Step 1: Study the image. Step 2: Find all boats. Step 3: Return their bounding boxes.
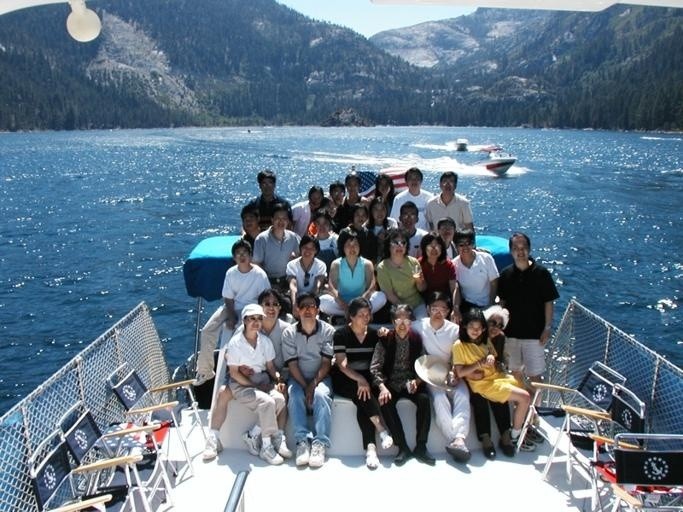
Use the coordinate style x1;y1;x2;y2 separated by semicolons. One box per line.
1;234;683;512
486;152;517;175
457;138;469;150
481;146;503;152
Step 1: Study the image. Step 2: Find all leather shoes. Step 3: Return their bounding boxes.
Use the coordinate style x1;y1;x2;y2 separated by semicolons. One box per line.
395;448;412;463
483;437;496;459
413;445;436;463
498;438;514;457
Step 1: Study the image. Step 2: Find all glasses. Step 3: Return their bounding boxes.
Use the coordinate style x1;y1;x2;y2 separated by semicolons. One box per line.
265;302;279;307
236;250;247;257
301;304;317;310
443;182;454;187
468;326;482;330
335;192;343;196
390;240;408;248
396;319;411;326
427;245;441;249
489;320;504;329
403;214;415;219
247;316;262;320
430;307;448;315
459;241;472;247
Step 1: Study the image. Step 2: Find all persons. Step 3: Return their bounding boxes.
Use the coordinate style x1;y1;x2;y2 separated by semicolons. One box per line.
376;228;428;321
417;231;463;323
337;202;378;265
398;201;429;260
390;167;435;231
318;226;388;325
368;174;396;216
425;171;474;232
192;239;272;387
313;209;341;274
202;288;293;461
330;296;394;469
451;307;545;453
362;196;399;262
369;303;437;464
376;291;472;462
341;174;371;224
247;169;294;232
290;185;324;239
285;234;327;319
306;197;338;235
328;182;350;235
252;203;302;293
464;304;515;458
280;290;336;468
240;204;263;252
450;226;500;316
225;302;285;466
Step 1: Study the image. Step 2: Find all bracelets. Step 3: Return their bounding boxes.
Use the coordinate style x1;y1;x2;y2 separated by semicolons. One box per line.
476;360;482;369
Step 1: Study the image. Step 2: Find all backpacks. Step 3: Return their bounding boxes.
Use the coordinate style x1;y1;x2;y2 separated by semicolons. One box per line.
494;232;561;406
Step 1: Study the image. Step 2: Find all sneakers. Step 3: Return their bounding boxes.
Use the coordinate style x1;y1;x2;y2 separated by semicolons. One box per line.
382;435;393;452
510;435;535;452
365;451;377;469
274;430;292;458
192;370;214;386
258;444;282;465
330;313;343;323
295;442;309;465
242;430;259;455
529;427;544;443
308;440;325;467
204;435;223;460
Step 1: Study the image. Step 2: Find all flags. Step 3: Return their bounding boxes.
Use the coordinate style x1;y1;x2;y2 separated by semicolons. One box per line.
356;170;409;197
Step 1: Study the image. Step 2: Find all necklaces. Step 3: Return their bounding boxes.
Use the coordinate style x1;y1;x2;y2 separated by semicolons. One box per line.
390;258;404;268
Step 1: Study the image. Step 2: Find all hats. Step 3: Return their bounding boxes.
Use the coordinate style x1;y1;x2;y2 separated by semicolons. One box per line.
241;303;267;318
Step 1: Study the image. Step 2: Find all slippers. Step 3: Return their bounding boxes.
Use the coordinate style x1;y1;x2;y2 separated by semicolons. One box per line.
445;440;472;463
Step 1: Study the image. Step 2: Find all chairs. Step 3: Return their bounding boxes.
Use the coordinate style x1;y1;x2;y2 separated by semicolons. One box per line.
563;383;646;512
105;361;208;468
610;433;683;512
28;428;143;512
56;401;177;512
514;361;628;476
